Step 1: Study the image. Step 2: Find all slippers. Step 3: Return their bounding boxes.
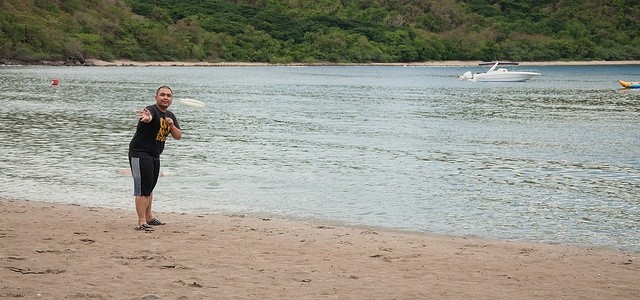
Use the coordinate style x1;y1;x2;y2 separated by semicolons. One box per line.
138;223;154;231
147;217;166;225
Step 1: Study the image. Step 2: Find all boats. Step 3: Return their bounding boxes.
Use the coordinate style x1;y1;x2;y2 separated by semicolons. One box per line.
618;80;640;88
458;62;542;82
178;95;208;110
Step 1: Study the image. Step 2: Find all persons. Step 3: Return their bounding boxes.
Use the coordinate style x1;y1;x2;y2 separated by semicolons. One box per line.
129;86;181;232
